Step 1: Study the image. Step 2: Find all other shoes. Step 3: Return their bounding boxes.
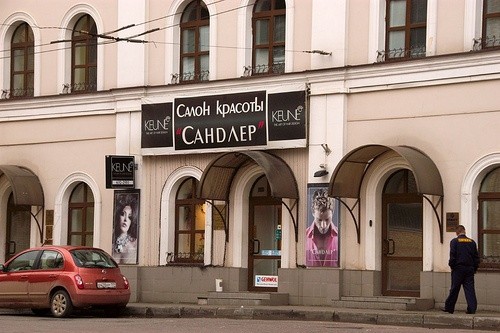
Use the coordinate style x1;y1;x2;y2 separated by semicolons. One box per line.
441;306;453;314
466;311;476;314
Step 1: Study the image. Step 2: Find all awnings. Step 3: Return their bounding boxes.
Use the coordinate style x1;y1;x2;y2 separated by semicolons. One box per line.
196;150;298;242
328;144;443;243
0;165;44;243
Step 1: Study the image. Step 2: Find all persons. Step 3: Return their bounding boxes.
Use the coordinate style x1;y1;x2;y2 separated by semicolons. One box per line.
440;225;478;314
112;202;138;254
305;190;338;266
46;255;55;266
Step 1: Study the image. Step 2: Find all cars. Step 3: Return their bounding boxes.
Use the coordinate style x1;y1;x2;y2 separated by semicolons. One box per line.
0;246;131;318
